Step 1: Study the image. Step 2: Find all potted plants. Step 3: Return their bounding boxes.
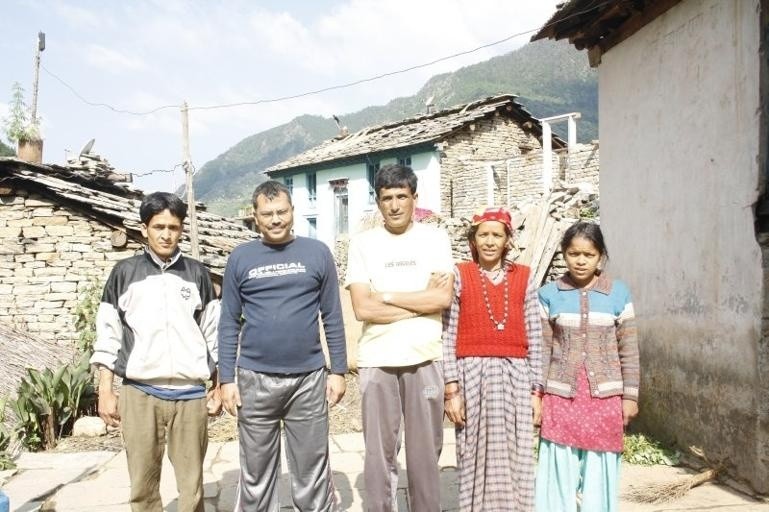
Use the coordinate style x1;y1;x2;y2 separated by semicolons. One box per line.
0;79;43;163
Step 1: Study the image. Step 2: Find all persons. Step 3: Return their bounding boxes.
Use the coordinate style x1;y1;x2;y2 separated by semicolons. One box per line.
219;181;347;512
345;163;454;512
90;190;224;511
442;202;543;511
534;223;641;511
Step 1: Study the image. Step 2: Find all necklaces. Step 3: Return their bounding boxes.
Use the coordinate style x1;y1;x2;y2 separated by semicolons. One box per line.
478;260;512;330
